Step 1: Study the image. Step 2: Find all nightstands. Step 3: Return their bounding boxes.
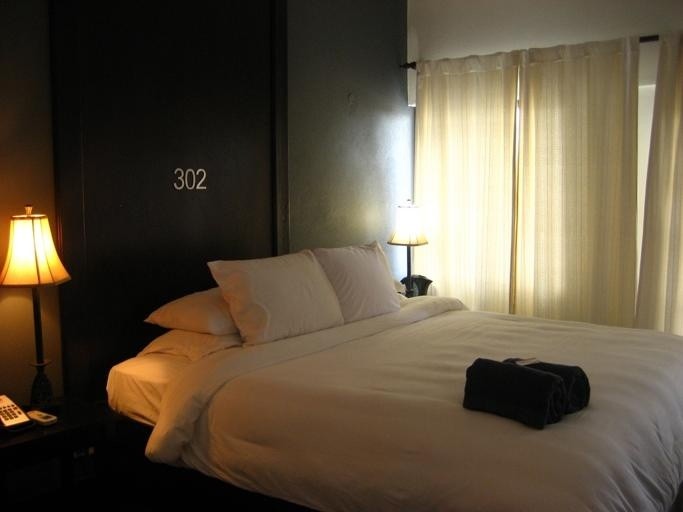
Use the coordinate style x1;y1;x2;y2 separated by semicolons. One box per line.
0;398;129;511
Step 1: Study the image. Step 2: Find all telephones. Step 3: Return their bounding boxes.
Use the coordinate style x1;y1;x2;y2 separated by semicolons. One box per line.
0;394;30;427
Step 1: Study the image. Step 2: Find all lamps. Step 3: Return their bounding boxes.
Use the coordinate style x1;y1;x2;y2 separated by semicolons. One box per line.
386;197;430;297
0;204;72;407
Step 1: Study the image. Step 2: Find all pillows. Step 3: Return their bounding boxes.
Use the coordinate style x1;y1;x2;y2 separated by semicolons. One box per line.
207;249;345;348
136;329;242;364
312;239;401;324
143;285;237;336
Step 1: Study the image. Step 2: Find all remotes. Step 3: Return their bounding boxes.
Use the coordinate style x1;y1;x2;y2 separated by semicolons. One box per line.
26;410;58;426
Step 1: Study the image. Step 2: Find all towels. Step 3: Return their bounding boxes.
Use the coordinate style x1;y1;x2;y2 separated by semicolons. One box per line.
463;358;590;429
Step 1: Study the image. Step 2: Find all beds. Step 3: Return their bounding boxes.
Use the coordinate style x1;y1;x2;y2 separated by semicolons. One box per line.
107;295;683;512
